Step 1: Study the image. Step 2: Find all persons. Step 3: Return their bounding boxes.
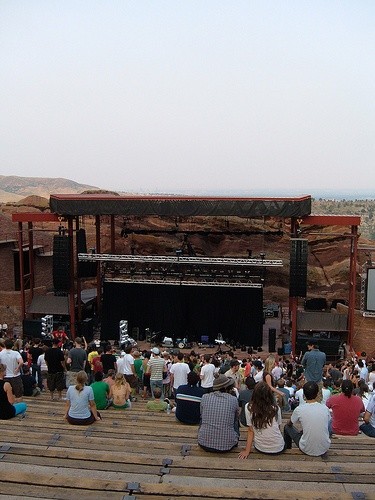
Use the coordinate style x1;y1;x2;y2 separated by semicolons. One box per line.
262;354;283;435
360;381;375;438
63;370;101;425
301;338;326;402
145;347;167;398
147;388;168;411
108;373;132;409
169;352;191;391
283;381;333;457
199;353;215;394
326;380;365;436
102;368;116;387
224;359;241;392
237;382;285;459
90;370;112;410
196;374;241;453
175;371;205;426
120;346;138;388
0;327;375;459
65;336;87;372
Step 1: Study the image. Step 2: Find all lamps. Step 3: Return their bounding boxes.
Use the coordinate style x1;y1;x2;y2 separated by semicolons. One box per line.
41;317;48;336
120;320;129;341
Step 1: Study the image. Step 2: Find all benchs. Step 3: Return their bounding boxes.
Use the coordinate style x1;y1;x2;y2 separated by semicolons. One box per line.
0;387;375;500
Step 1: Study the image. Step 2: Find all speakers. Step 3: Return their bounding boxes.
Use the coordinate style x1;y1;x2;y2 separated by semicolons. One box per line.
52;235;73;291
288;238;308;297
269;328;276;353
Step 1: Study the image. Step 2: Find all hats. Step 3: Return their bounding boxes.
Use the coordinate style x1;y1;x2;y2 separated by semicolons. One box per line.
230;361;240;366
151;347;160;354
212;374;235;390
252;360;263;368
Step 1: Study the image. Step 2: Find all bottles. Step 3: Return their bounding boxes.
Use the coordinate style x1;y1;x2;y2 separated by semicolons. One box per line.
167;404;170;415
127;399;131;408
